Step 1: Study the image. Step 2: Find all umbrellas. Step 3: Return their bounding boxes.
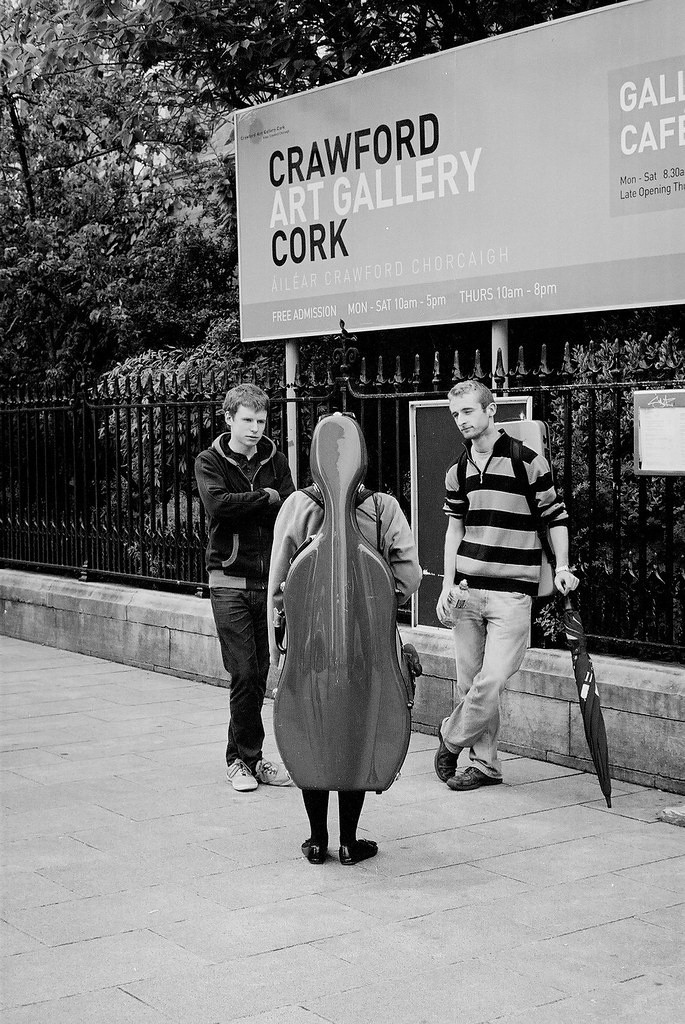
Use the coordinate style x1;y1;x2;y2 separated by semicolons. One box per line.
561;584;612;809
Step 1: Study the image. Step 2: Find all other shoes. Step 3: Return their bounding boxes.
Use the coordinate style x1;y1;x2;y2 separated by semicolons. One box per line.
339;839;379;866
301;838;328;863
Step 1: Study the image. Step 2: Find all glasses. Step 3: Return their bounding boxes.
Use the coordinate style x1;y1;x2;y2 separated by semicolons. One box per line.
318;412;356;421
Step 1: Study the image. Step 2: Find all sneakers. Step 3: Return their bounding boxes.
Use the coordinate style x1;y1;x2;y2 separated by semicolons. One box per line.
447;763;503;791
254;756;293;787
226;758;258;791
434;724;459;782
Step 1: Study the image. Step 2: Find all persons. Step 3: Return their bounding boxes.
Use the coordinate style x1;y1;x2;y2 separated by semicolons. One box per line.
266;412;421;864
194;384;297;791
435;380;579;791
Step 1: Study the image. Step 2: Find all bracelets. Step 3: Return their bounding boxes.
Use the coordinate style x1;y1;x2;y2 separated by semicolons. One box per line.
555;565;570;573
